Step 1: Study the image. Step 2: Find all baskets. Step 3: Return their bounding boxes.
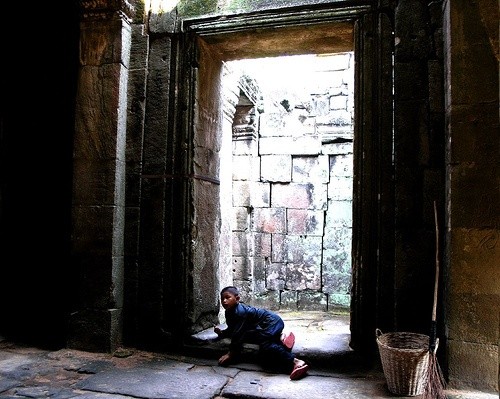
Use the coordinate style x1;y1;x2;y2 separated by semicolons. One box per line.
376;327;439;397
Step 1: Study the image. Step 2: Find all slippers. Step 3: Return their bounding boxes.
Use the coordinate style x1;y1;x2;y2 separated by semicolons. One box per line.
283;331;295;349
290;363;308;379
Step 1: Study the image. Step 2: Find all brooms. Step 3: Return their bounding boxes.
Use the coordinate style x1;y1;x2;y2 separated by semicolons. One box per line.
408;200;454;399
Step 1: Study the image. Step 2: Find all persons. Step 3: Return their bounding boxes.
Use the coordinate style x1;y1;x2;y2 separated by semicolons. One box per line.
213;286;308;380
